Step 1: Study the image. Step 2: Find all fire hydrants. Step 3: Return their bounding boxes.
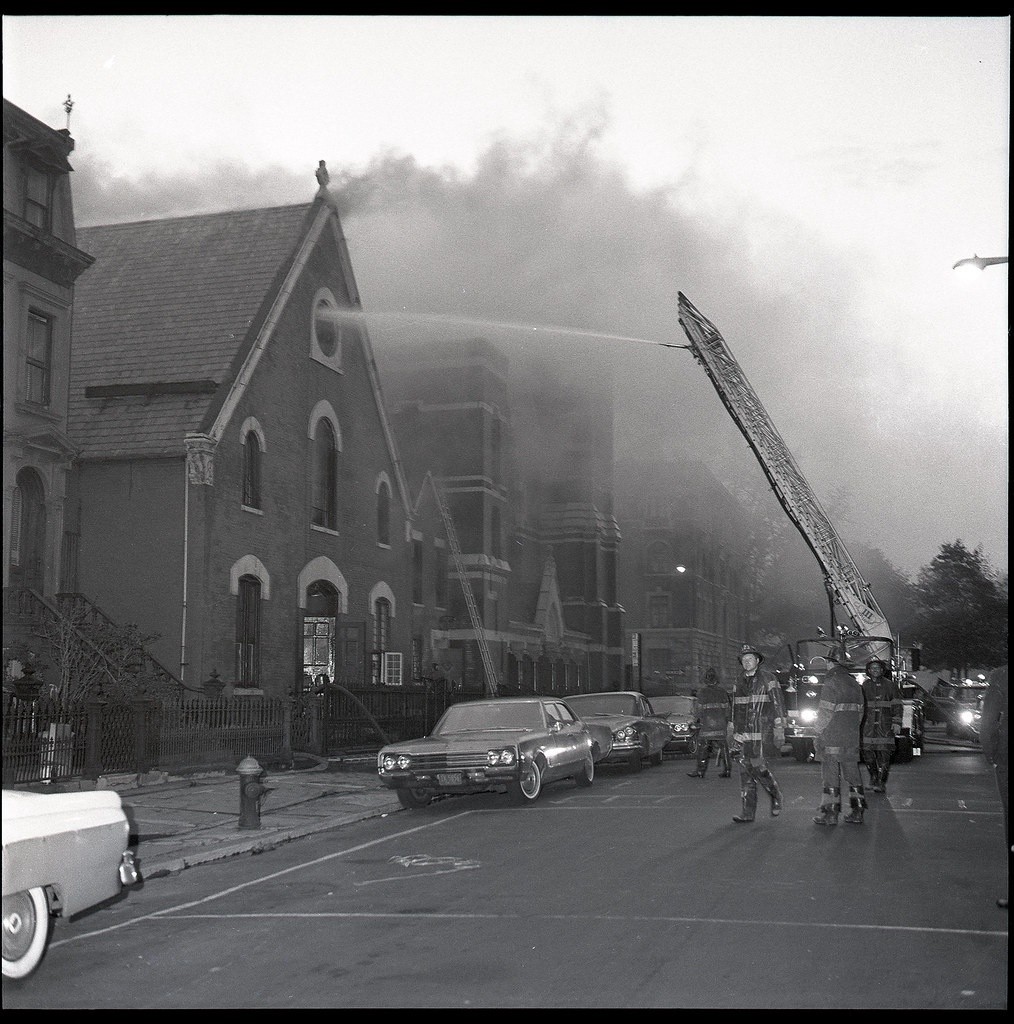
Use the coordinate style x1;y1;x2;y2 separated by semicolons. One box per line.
237;754;269;831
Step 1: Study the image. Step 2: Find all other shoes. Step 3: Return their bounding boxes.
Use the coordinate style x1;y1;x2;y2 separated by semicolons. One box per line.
996;899;1008;907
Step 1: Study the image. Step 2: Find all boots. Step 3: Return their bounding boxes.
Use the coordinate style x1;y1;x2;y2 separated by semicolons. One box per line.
865;760;878;790
873;759;891;793
686;758;708;778
732;782;758;822
843;786;868;823
813;787;841;825
719;755;732;777
763;780;784;816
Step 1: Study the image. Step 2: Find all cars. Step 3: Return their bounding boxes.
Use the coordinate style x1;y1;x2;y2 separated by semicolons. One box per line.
561;692;670;773
376;697;613;815
0;785;139;985
944;680;989;738
645;696;703;756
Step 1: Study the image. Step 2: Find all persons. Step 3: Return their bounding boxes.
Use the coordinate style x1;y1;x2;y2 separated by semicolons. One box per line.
813;646;866;825
687;664;733;778
860;657;904;794
728;642;782;822
980;664;1008;910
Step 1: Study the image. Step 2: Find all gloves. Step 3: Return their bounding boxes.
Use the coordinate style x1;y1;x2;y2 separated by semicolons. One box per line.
891;723;901;734
773;733;785;748
813;735;825;751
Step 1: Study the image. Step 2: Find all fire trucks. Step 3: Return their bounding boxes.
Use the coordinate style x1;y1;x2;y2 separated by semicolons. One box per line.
657;292;927;766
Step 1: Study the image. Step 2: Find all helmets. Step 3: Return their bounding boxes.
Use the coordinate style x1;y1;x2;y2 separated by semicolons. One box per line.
821;646;854;667
738;643;764;666
866;657;887;676
